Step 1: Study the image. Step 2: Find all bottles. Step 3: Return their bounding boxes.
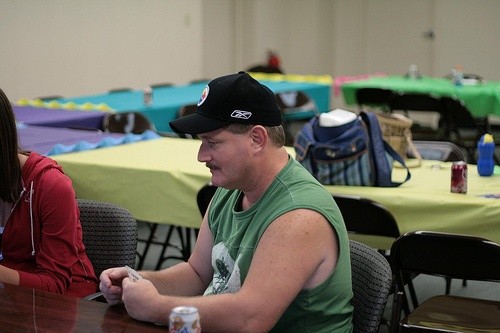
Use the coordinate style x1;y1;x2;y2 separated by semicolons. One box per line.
477;134;495;176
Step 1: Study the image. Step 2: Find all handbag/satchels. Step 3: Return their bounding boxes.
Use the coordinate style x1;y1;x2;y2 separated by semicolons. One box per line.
365;110;423;168
294;111;411;188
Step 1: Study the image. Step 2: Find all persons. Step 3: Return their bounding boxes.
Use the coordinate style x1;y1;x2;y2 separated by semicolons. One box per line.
99;71;354;333
0;89;97;300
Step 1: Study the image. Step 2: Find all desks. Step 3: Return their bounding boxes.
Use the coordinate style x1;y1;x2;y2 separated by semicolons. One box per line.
17;73;332;136
12;106;160;157
341;75;500;117
0;280;169;333
48;137;500;262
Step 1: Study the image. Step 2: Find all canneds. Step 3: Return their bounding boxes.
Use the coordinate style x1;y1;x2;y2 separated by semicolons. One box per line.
407;64;418;80
168;306;201;333
450;161;468;194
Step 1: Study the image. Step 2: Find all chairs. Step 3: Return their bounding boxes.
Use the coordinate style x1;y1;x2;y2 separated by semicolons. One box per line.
406;141;467;295
354;88;500;166
330;194;420;309
74;198;138;303
391;230;500;333
98;112;184;270
196;182;218;219
348;240;393;333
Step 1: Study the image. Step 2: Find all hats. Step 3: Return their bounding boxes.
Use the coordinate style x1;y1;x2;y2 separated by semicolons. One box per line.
169;71;283;135
